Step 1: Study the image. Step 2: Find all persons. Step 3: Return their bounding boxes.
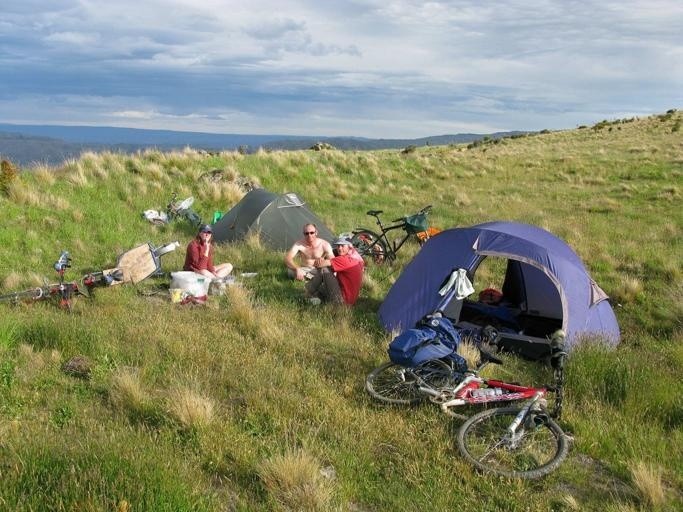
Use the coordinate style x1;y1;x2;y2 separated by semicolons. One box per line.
184;224;234;295
302;238;364;309
284;224;335;282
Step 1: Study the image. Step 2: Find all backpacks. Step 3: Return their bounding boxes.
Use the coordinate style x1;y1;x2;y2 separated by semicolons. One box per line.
386;315;457;366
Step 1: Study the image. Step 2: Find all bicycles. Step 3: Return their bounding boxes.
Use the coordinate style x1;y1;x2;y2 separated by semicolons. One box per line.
165;192;201;237
348;205;432;269
365;328;567;480
0;251;123;314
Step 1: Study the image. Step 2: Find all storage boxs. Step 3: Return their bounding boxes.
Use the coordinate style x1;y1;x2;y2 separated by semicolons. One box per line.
169;269;212;296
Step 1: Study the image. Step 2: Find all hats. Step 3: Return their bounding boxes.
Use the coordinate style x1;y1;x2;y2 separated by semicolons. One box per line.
199;225;212;234
332;234;351;245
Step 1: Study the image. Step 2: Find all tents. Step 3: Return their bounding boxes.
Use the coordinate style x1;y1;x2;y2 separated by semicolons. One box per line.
211;188;339;255
376;220;621;371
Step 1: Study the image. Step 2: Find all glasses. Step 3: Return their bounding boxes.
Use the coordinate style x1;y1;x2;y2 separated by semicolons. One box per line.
305;230;314;237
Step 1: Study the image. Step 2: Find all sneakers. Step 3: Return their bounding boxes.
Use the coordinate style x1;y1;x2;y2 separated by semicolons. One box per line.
301;296;321;305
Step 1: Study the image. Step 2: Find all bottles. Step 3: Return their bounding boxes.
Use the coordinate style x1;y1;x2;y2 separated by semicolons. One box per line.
454;375;482;400
470;386;511;397
